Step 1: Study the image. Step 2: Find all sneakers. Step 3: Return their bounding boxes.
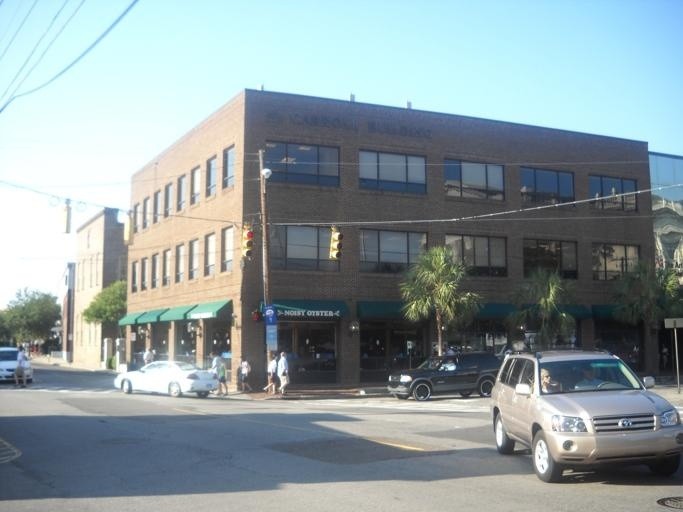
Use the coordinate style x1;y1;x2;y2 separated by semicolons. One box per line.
224;393;229;395
263;388;268;392
15;384;19;386
273;391;277;395
282;392;287;395
21;385;26;388
241;390;246;393
215;392;221;395
249;389;253;391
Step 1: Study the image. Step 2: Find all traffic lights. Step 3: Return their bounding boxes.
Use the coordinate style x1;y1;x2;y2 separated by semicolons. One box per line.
240;225;255;257
327;227;343;261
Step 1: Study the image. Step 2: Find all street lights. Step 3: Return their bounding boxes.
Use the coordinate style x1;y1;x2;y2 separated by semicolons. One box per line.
257;167;277;397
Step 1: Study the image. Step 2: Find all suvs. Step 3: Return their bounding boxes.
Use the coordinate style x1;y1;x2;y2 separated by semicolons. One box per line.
487;345;682;486
384;349;502;402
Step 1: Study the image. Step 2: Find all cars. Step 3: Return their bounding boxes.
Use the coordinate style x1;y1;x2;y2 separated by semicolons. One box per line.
0;345;34;385
113;359;219;400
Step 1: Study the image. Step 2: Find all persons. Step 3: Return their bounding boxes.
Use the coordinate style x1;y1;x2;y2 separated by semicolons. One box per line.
143;348;153;365
239;355;251;393
209;351;222;395
572;366;605;390
277;351;288;394
152;347;159;362
661;343;670;369
261;354;278;394
530;367;560;394
13;346;27;387
216;358;228;396
441;359;456;371
